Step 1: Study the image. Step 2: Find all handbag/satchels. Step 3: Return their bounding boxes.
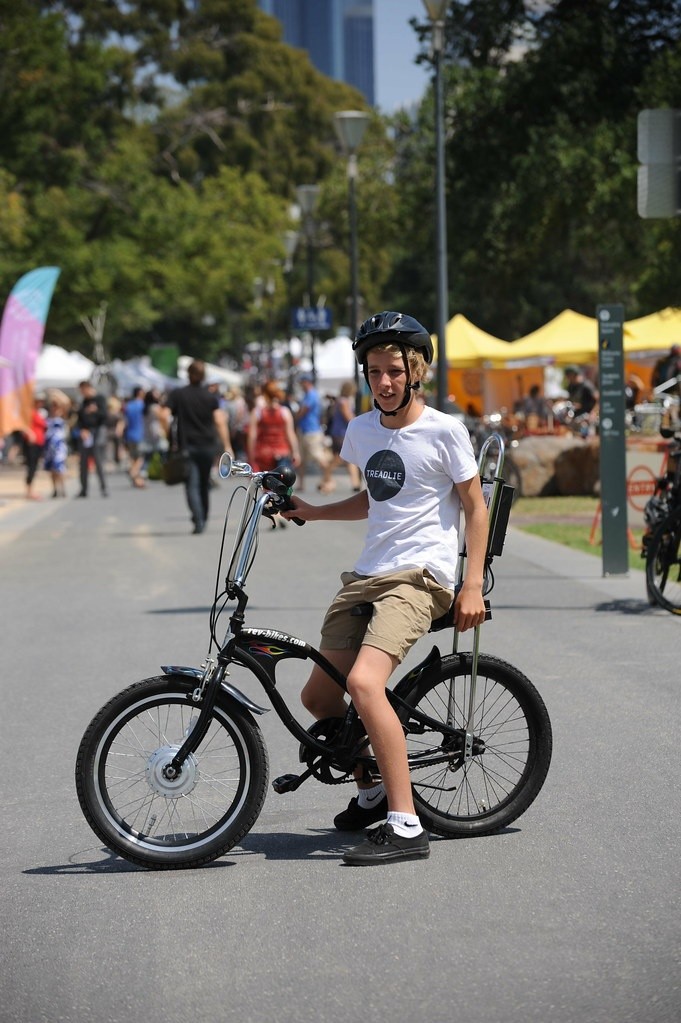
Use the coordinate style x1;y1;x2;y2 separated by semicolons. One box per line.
162;449;191;485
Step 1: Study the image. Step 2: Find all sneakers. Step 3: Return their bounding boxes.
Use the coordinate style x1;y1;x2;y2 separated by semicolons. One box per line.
333;795;385;830
343;823;430;866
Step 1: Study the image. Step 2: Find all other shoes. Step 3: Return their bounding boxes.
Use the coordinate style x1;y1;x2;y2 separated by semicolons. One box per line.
194;520;204;534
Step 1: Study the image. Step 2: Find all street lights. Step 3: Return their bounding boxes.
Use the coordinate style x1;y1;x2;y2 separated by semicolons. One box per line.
331;110;370;415
280;186;324;388
423;1;455;414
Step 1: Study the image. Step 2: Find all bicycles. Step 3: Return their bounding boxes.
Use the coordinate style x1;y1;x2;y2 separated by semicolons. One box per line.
74;433;555;869
639;430;681;615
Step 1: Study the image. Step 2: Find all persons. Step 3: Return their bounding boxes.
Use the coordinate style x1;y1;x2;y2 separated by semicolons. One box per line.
281;312;489;866
650;345;681;408
0;372;363;500
157;361;235;533
514;385;545;414
564;365;597;416
248;381;301;530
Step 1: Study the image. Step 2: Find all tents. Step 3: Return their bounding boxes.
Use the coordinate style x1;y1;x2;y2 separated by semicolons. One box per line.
289;336;366;388
30;344;243;393
423;307;681;417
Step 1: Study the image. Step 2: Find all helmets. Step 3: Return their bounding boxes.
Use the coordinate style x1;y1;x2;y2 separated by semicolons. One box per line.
352;312;433;365
